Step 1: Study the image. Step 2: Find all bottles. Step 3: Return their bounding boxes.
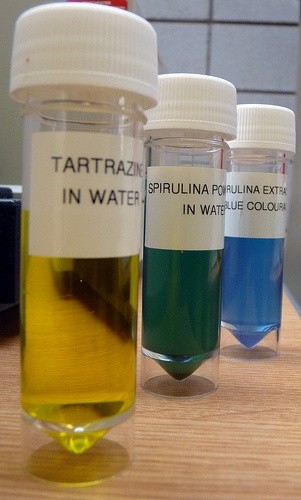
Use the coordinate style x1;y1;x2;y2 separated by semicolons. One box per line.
222;104;296;360
127;66;237;397
9;0;161;487
